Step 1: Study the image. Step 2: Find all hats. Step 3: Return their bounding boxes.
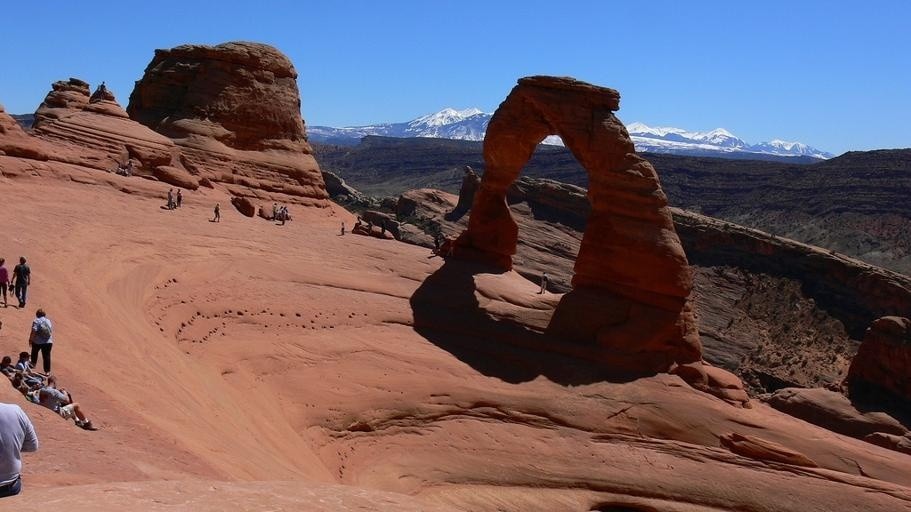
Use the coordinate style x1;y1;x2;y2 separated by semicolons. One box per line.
19;352;31;357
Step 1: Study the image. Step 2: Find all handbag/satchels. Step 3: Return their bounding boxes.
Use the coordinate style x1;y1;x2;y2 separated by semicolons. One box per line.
8;283;15;293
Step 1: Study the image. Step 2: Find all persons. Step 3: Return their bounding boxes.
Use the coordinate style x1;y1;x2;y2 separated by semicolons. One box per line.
168;188;182;209
0;308;97;431
357;216;386;236
434;238;440;256
0;401;38;497
341;223;344;235
541;273;547;294
0;257;31;307
273;203;288;225
214;203;220;222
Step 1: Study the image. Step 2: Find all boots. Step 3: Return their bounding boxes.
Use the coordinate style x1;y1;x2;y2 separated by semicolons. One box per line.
75;421;98;431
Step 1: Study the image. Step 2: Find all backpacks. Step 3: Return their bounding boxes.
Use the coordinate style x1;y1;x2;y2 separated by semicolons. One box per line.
544;277;547;281
38;320;50;340
